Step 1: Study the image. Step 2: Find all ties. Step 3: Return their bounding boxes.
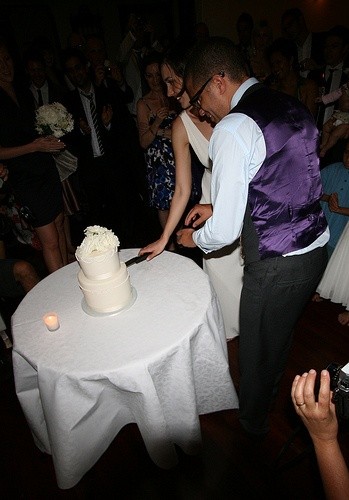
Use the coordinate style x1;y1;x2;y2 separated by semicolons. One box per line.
80;91;104;155
36;89;44;107
317;68;339;131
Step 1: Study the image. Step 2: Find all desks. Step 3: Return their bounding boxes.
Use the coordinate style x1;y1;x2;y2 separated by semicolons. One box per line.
10;249;240;490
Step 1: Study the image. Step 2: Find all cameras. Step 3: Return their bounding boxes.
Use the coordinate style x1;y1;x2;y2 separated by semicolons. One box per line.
325;362;349;416
103;64;111;75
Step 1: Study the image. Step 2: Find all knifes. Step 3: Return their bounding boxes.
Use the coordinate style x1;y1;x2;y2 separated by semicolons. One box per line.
125;252;153;267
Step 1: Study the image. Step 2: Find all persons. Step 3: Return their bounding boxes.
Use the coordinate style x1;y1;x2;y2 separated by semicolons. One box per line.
0;22;349;369
290;367;349;500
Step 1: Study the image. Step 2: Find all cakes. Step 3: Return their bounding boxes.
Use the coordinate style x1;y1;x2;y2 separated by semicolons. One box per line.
75;225;133;313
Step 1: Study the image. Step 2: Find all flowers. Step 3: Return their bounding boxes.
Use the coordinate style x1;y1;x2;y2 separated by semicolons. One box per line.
36;103;73;141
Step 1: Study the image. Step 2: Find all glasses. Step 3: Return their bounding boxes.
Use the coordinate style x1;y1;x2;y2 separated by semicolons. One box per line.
189;72;225;110
